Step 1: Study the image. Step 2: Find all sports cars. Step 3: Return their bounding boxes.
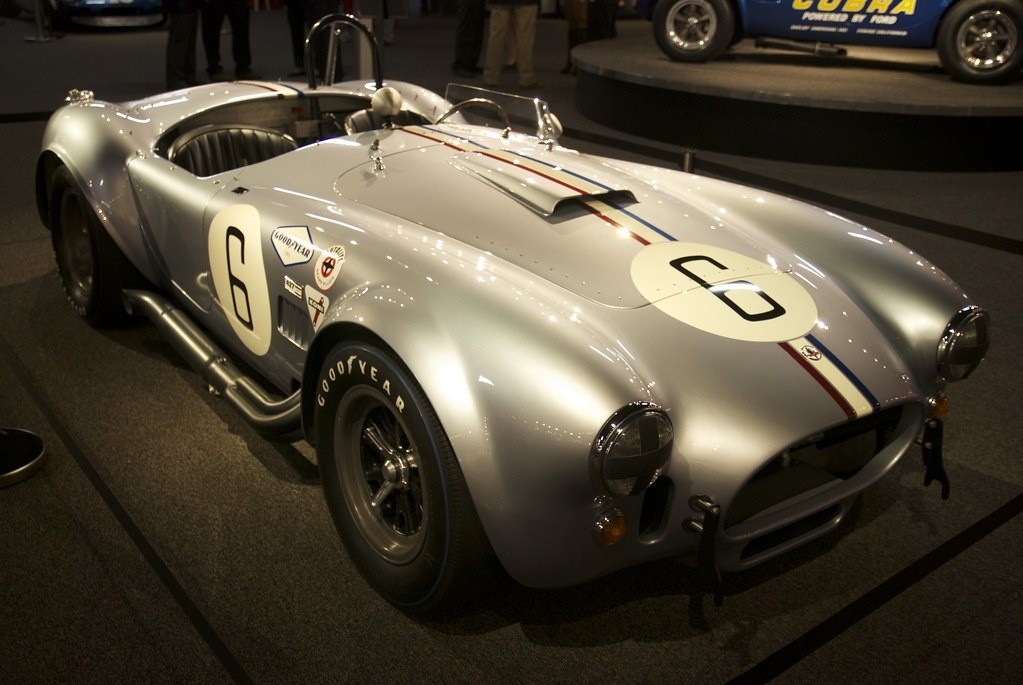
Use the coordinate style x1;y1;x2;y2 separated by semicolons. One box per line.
33;9;989;624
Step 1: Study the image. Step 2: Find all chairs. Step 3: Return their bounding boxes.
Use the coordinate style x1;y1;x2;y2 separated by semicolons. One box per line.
344;106;434;135
165;122;299;178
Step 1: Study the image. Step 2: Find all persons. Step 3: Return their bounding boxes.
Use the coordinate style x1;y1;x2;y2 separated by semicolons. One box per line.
159;0;257;96
452;0;618;96
284;1;329;78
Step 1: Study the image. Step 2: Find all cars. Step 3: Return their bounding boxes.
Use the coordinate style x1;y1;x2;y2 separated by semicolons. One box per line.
599;0;1023;87
